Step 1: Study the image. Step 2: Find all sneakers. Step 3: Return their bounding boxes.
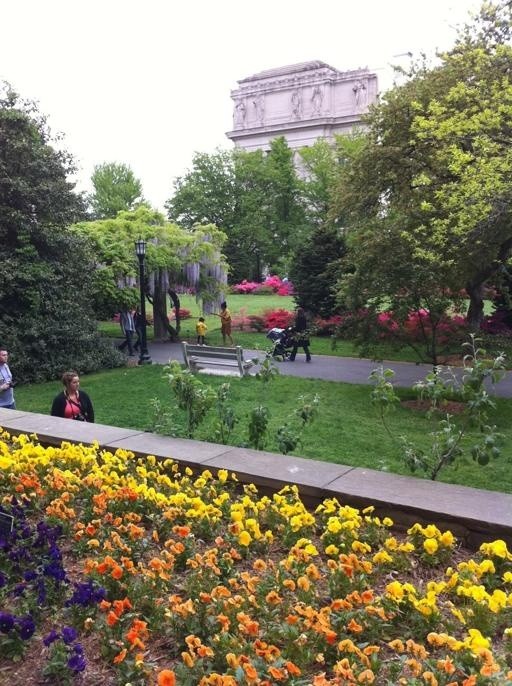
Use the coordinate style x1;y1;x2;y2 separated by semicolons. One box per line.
118;344;138;355
287;355;294;360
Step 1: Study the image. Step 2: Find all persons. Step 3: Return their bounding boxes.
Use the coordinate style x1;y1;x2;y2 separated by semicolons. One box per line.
233;79;369;129
0;347;18;410
131;304;152;353
117;308;137;356
210;301;234;348
284;307;312;362
51;370;95;425
195;317;209;347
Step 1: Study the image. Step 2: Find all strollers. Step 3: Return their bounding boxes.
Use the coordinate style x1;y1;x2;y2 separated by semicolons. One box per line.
266;327;294;362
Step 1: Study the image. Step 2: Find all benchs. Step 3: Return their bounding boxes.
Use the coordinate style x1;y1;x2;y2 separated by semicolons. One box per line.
181;341;253;378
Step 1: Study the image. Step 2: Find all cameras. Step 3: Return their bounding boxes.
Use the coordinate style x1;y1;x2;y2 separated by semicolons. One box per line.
8;379;19;389
74;412;85;421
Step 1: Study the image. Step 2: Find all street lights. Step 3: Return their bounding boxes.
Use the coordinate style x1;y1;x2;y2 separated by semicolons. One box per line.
134;235;152;365
